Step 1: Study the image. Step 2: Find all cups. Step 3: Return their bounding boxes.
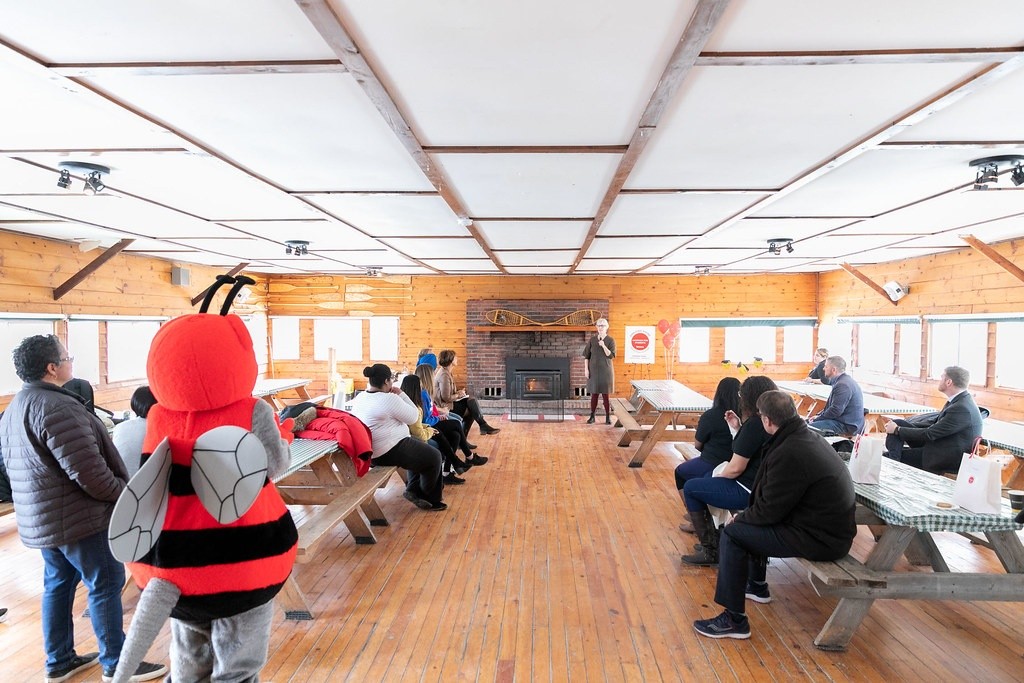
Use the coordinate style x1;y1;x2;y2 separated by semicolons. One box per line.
1008;489;1024;512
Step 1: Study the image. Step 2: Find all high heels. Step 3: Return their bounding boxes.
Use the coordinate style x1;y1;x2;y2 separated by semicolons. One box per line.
458;441;477;450
480;423;500;435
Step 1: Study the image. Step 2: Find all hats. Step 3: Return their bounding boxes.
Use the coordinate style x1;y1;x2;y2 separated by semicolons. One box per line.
596;318;608;326
416;354;437;371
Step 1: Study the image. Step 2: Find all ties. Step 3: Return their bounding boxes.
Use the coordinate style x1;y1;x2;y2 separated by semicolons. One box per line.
940;401;949;413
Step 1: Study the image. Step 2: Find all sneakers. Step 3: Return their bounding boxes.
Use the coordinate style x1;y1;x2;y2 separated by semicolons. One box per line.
102;657;167;683
428;500;447;511
742;579;772;604
48;652;101;683
443;473;466;484
465;453;488;466
403;485;432;509
694;609;751;640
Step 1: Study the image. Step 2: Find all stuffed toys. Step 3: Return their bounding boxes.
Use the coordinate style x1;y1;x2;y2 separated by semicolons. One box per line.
107;314;298;683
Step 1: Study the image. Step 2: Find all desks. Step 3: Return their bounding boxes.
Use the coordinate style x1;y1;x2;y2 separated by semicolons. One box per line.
616;377;714;467
79;437;389;620
251;378;313;410
769;380;1024;650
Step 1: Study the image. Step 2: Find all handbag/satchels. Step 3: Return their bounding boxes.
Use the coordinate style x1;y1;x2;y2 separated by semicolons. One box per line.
952;437;1001;515
822;435;854;461
848;420;883;485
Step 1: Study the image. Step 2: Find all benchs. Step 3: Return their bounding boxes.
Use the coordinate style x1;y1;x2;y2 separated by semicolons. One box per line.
278;466;400;563
674;441;888;598
274;394;332;419
610;397;642;442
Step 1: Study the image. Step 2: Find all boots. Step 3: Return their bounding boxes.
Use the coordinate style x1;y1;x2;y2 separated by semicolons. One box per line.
587;413;596;423
606;414;611;424
680;507;720;569
447;448;472;475
677;489;699;537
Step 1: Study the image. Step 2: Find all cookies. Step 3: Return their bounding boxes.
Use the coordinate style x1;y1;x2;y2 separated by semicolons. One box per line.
936;502;951;508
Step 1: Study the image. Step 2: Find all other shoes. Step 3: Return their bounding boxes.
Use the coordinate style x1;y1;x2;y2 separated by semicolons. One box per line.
0;608;9;623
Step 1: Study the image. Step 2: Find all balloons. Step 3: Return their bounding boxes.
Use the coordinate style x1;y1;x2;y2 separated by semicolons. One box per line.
662;333;674;350
658;319;669;334
669;321;680;337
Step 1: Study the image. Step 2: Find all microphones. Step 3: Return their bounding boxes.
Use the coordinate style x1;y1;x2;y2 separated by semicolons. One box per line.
599;334;602;340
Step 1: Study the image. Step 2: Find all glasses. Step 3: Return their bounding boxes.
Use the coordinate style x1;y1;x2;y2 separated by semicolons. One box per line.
757;412;763;418
738;390;742;398
388;374;395;380
57;352;74;363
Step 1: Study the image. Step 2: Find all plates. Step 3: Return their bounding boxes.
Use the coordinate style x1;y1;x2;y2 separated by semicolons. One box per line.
930;502;960;510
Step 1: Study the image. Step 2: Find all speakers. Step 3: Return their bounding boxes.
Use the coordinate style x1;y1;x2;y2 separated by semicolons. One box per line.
171;267;191;286
233;288;252;304
883;281;904;302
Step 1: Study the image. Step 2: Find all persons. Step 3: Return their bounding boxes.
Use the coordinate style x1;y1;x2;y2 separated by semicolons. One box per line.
692;391;856;639
401;347;499;486
0;334;168;683
680;376;779;567
884;366;982;474
806;356;864;436
803;347;831;384
674;377;743;533
111;386;158;479
350;364;447;511
582;318;616;425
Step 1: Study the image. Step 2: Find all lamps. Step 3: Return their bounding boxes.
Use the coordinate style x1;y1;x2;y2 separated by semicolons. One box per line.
284;240;309;256
969;155;1024;190
695;266;712;275
56;159;111;195
365;267;382;277
768;238;794;255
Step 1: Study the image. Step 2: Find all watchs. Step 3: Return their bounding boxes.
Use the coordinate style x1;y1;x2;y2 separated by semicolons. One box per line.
894;427;899;436
